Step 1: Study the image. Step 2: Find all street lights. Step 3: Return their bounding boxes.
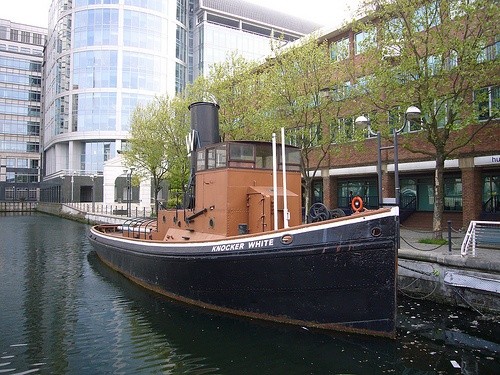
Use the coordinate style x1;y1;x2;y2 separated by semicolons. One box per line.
90;174;98;212
353;106;421;248
122;166;136;218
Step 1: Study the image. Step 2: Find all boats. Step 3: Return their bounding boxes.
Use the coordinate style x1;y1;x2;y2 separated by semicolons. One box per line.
88;99;402;341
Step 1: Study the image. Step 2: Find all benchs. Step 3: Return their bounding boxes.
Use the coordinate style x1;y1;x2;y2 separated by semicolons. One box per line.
101;226;118;233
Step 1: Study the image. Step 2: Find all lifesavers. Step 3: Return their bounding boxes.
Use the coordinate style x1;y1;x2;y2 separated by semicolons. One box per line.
352;196;363;211
